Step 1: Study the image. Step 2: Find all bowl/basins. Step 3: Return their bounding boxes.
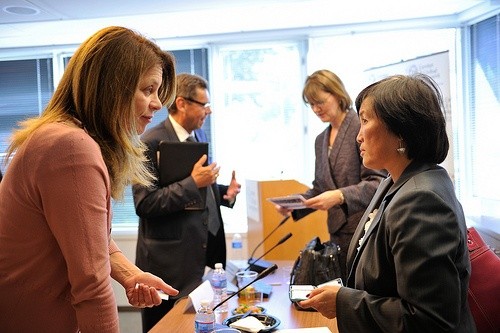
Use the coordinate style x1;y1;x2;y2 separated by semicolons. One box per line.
211;328;241;333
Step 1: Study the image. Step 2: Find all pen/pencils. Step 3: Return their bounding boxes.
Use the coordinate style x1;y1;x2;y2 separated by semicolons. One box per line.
270;283;281;286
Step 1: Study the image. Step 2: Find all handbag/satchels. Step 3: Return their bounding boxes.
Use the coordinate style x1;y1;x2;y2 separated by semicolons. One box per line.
289;236;343;312
467;226;500;333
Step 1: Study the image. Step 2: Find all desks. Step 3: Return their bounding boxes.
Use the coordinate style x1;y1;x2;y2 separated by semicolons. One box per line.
145;258;339;333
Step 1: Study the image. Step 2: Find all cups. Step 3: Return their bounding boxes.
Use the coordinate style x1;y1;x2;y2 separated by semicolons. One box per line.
245;283;263;307
236;271;258;307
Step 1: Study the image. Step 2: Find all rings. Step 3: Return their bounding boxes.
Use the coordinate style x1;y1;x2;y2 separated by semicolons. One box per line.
215;174;218;178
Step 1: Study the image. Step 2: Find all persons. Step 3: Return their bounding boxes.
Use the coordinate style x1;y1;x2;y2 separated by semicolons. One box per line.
296;76;473;333
132;72;240;333
275;70;389;278
0;26;179;333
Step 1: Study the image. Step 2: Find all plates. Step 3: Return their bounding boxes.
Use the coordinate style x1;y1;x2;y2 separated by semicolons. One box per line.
222;313;281;333
231;307;266;315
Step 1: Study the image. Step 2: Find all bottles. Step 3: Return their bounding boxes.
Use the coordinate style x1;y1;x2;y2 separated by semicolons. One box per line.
231;233;242;259
194;301;217;333
211;263;228;313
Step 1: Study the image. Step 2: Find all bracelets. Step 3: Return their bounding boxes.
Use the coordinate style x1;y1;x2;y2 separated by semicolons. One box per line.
335;189;345;206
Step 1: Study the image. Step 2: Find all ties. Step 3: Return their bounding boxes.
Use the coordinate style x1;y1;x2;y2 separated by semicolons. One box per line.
186;136;220;236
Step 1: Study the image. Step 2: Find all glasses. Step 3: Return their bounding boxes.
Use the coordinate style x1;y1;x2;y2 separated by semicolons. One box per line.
176;96;210;107
308;93;333;108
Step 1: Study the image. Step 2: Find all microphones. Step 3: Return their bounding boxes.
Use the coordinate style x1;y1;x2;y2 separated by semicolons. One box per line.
248;215;290;272
237;233;292;298
212;264;278;311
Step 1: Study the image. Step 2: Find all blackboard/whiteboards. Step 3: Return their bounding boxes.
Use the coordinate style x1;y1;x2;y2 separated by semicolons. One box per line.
364;50;452;191
